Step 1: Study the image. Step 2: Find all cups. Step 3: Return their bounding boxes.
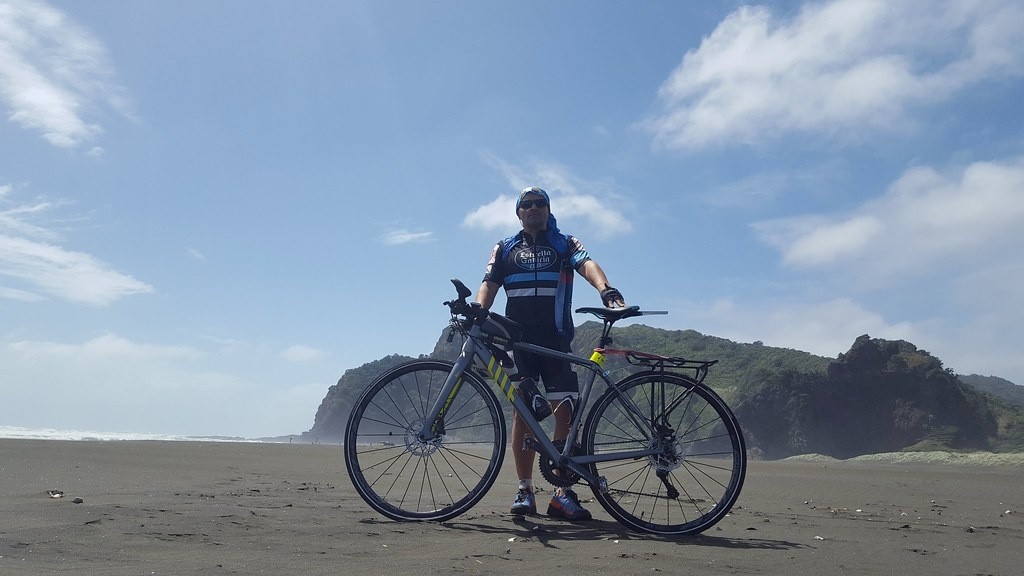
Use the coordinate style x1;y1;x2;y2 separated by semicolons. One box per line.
521;378;553;423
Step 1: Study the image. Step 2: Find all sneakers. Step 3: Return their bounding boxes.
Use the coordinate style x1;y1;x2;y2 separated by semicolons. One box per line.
510;488;537;516
547;491;591;522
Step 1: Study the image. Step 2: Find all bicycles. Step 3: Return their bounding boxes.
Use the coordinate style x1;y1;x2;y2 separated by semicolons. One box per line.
342;279;748;536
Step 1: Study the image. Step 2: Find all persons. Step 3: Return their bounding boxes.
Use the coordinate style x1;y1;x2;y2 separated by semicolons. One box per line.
468;187;625;520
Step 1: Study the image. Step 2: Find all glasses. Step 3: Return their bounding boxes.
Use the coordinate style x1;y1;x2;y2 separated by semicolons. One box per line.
520;199;546;209
